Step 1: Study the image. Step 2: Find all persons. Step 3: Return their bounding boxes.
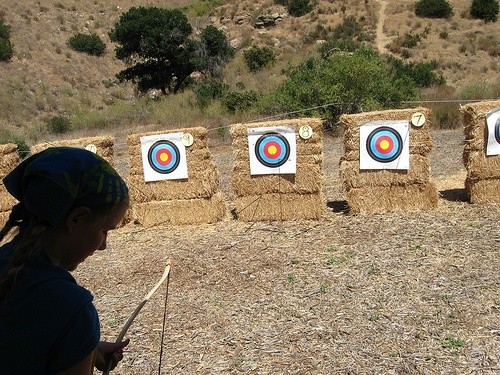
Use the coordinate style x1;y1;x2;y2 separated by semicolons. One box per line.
0;146;131;375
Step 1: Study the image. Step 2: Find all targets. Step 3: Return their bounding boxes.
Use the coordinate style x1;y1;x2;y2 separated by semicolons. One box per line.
140;132;190;184
359;119;410;171
246;126;298;176
485;108;500;157
